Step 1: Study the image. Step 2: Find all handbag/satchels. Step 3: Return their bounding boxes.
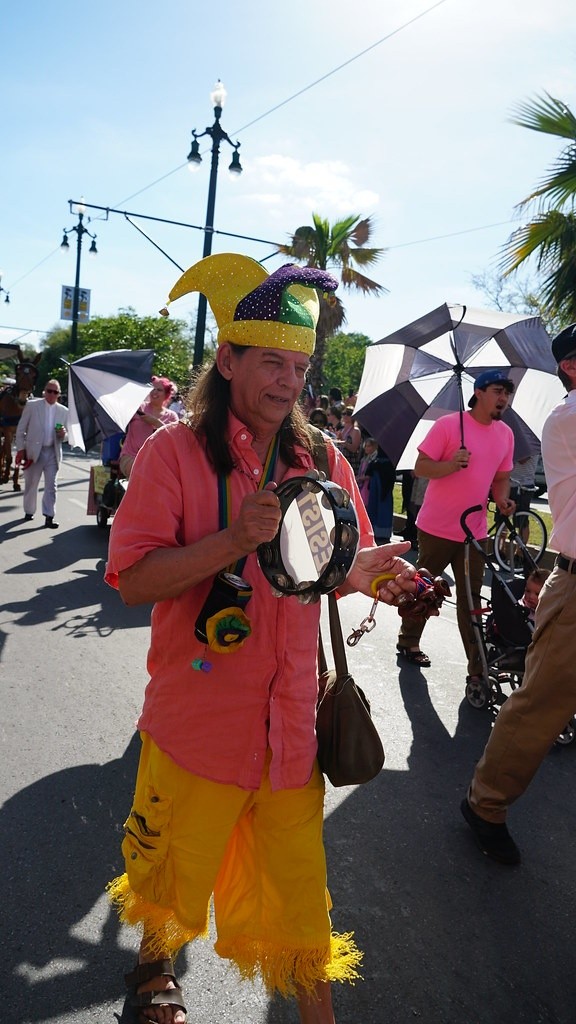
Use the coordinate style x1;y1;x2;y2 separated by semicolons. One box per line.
316;669;384;788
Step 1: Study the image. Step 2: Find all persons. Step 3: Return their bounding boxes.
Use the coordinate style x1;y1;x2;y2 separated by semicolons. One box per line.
103;254;417;1024
304;388;430;550
524;569;552;620
488;453;540;564
166;383;196;418
15;380;69;528
396;370;516;682
460;323;576;864
118;376;179;482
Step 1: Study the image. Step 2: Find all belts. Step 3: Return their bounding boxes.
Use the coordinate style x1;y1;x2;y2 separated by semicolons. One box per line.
558;553;576;574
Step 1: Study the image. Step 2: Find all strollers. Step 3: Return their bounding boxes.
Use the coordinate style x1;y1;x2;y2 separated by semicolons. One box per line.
459;503;576;748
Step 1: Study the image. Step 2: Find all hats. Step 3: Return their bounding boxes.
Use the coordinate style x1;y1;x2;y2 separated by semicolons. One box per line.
467;370;508;409
552;322;576;364
166;253;339;356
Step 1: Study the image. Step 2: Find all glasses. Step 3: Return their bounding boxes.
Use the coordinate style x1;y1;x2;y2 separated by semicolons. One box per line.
314;419;325;424
46;388;60;395
342;412;352;417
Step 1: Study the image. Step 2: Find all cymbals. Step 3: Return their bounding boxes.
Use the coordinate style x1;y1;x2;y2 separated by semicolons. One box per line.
256;469;359;606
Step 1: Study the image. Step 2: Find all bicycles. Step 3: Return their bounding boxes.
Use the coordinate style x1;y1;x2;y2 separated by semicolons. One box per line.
488;470;547;573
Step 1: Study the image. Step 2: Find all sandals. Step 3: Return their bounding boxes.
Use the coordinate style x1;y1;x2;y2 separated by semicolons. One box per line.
123;945;187;1024
398;641;431;665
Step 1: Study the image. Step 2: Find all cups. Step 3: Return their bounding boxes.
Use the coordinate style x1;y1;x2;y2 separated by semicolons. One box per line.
56;424;63;431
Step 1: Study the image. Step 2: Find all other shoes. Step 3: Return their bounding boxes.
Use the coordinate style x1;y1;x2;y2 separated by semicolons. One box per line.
498;557;525;573
45;517;59;528
461;797;521;868
404;539;419;552
486;550;507;564
394;528;408;537
26;513;34;521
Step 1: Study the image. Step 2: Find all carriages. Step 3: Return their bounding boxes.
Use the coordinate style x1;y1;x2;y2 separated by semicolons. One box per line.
0;343;43;492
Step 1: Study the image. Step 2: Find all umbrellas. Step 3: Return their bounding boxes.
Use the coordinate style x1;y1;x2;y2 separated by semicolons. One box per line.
351;302;568;471
60;348;156;457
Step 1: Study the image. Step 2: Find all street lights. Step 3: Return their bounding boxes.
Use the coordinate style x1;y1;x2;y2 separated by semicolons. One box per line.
61;196;99;362
187;77;242;375
